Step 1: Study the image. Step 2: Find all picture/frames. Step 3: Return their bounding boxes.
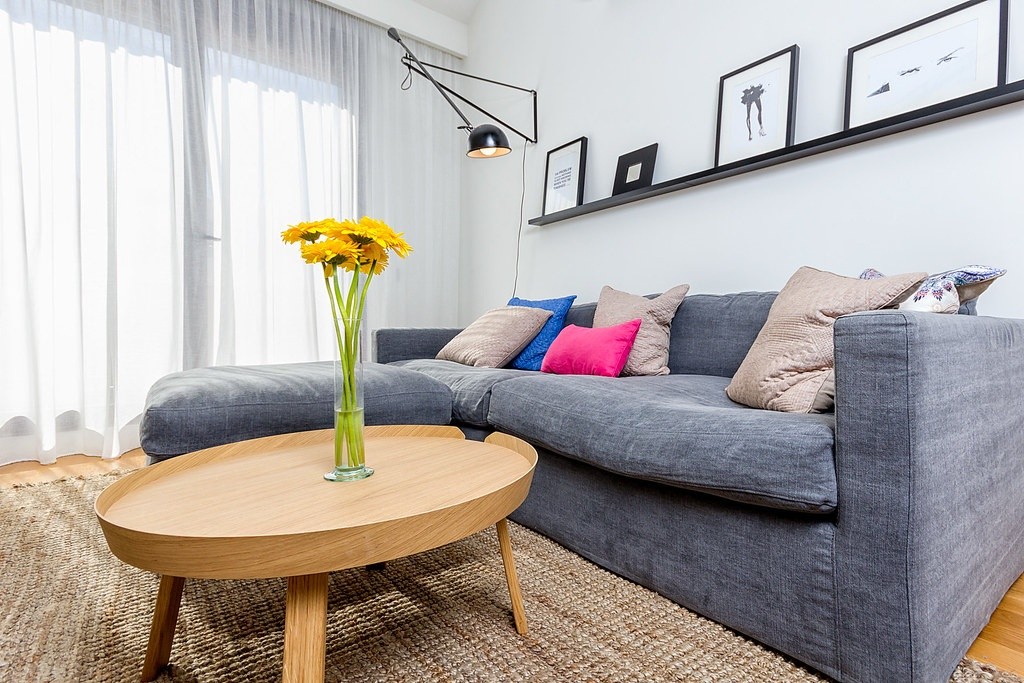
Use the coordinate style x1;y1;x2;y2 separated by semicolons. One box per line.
844;0;1008;131
542;136;588;216
714;44;800;168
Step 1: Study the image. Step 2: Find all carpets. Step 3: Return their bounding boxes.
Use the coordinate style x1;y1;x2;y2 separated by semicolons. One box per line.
0;465;1024;683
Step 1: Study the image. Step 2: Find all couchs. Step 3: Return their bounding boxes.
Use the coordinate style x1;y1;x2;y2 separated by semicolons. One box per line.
140;294;1023;683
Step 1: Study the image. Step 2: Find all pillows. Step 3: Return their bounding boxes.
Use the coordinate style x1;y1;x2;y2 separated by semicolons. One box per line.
592;284;690;376
506;295;578;371
857;264;1007;315
435;305;554;368
540;318;642;378
725;265;929;413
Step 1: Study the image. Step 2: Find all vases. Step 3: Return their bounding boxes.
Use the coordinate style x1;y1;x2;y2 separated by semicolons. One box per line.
323;318;378;483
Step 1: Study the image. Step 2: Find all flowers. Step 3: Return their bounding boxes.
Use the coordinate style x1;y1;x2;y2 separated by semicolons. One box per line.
280;216;413;318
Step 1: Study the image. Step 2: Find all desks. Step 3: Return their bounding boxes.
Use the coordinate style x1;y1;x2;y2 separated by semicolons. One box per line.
93;424;540;683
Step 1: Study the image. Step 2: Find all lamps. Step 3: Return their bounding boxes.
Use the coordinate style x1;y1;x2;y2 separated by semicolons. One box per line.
387;27;537;159
